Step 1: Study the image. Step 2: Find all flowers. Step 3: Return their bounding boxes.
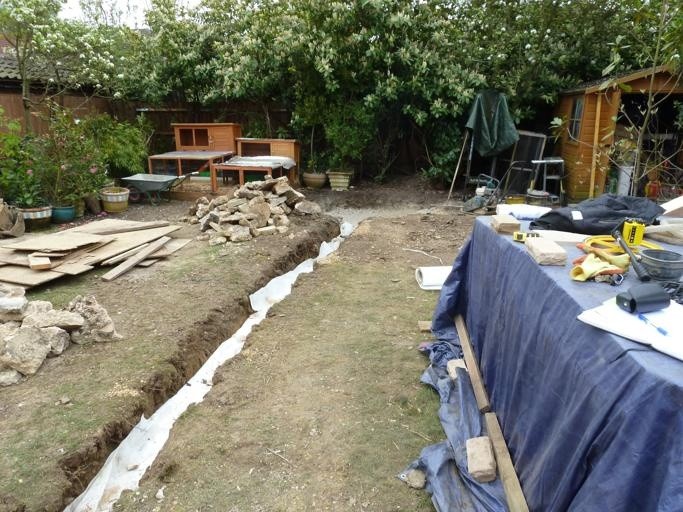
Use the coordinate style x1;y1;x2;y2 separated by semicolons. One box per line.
24;109;99;209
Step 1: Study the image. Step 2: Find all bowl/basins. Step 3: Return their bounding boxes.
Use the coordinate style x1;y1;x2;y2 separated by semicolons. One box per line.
639;249;682;279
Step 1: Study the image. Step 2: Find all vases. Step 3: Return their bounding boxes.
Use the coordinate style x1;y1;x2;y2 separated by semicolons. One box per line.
53;205;76;223
99;186;131;213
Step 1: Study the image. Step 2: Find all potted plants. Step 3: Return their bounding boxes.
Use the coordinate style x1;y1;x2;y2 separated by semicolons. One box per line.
286;94;380;194
1;109;52;232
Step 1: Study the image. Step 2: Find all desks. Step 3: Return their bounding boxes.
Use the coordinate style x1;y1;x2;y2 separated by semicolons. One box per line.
474;214;682;512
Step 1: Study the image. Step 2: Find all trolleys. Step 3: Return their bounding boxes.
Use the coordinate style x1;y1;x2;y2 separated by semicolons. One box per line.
121;171;200;206
